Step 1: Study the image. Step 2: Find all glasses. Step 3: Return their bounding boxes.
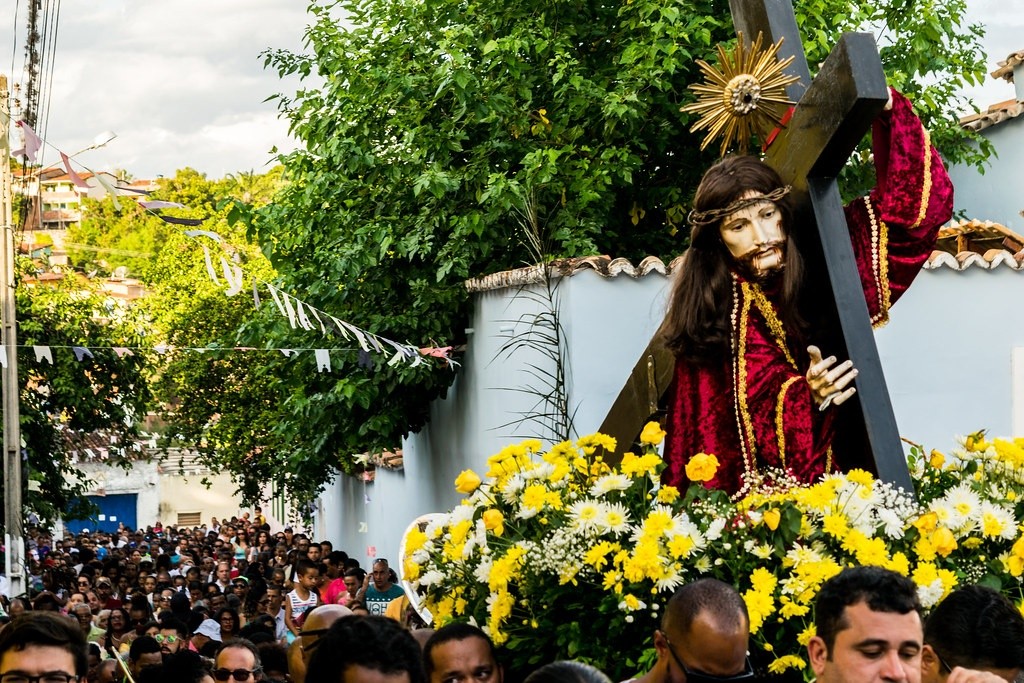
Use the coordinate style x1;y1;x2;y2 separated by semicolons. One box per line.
210;664;260;681
233;584;247;589
660;632;755;682
101;618;109;622
276;536;285;539
160;595;173;601
78;582;89;586
137;576;147;580
0;671;79;683
373;559;388;566
155;633;183;643
258;599;269;606
131;616;149;626
206;561;213;564
299;544;310;546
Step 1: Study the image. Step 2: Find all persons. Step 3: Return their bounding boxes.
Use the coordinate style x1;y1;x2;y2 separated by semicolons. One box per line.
806;565;925;683
522;660;612;683
0;504;436;683
662;86;956;502
923;584;1024;683
424;622;504;683
617;577;756;683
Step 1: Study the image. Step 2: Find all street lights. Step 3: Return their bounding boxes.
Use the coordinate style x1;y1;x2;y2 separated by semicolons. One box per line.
0;129;118;598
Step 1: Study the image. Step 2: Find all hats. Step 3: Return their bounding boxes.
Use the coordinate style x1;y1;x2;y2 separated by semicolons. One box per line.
193;618;223;643
232;575;249;586
284;526;293;533
